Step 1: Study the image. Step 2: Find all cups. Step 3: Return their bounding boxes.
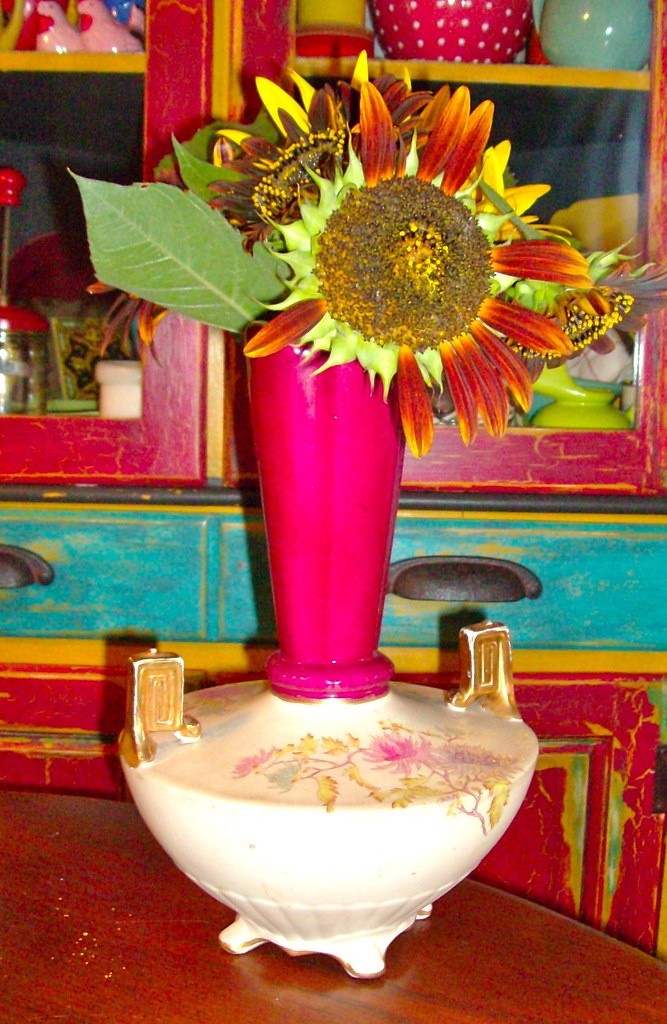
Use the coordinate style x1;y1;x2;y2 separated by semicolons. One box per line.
526;16;549;65
296;0;368;30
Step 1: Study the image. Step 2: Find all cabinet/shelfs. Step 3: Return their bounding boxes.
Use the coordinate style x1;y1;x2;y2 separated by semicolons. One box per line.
0;0;666;962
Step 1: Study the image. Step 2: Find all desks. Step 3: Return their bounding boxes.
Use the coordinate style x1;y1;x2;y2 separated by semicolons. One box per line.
0;789;667;1024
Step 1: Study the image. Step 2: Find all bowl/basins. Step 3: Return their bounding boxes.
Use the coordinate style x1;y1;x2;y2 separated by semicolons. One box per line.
368;0;534;65
533;0;652;70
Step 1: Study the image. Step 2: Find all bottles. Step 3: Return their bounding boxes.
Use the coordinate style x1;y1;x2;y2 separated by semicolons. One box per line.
0;305;49;420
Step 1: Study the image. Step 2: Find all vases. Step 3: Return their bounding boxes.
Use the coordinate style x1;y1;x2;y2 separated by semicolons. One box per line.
119;318;540;979
